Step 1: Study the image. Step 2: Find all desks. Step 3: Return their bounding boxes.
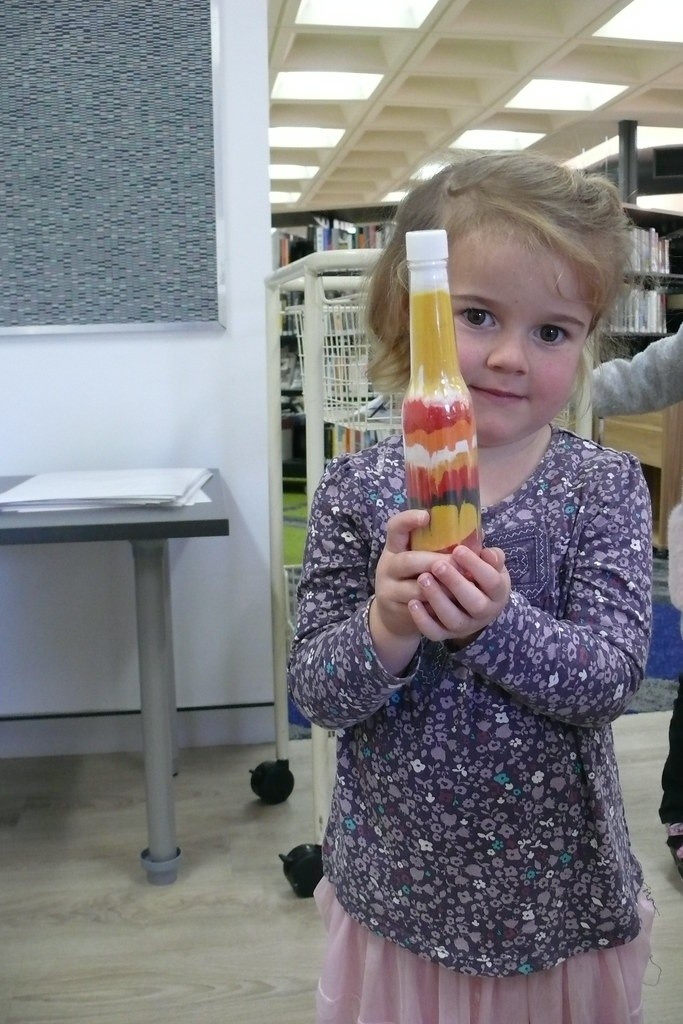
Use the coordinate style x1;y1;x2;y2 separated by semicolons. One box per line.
0;467;231;884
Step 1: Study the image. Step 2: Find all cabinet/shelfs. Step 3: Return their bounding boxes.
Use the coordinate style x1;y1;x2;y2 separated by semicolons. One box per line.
268;120;683;494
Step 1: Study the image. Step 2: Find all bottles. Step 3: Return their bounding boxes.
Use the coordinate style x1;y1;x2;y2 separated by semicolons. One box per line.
401;230;482;598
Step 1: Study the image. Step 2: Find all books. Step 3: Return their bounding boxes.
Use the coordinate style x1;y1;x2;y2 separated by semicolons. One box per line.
600;226;671;362
274;223;395;388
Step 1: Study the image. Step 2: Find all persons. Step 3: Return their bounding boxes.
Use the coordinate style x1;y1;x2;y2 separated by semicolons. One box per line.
286;154;662;1024
569;324;683;877
